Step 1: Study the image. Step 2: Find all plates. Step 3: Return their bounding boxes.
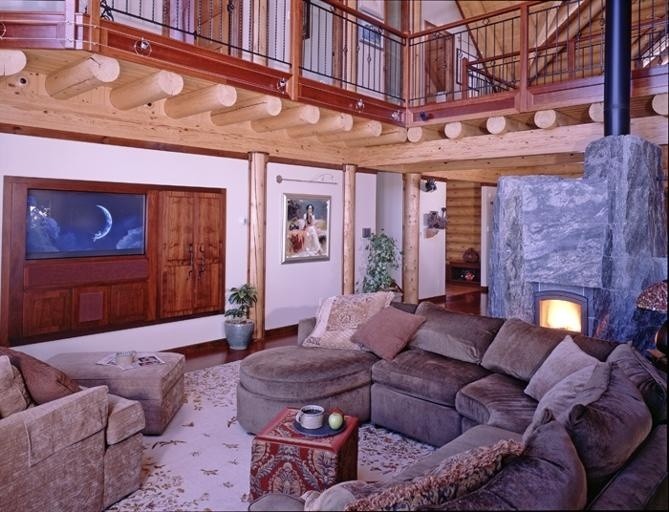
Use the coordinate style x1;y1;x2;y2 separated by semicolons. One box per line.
293;413;346;437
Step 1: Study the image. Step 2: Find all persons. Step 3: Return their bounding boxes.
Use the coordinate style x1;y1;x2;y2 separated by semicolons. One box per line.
296;203;323;257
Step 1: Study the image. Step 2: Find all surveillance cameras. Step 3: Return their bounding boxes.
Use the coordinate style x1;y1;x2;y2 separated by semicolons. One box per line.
425;178;436;192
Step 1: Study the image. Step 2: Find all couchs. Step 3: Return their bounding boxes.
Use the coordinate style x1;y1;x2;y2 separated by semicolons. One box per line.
237;293;418;435
43;351;186;436
454;317;632;434
0;346;146;511
249;345;669;511
371;311;507;447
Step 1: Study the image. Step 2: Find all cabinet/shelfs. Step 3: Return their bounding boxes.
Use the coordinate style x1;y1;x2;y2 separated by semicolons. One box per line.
158;187;225;322
1;175;157;348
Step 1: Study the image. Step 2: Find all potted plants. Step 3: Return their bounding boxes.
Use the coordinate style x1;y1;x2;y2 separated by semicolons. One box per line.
225;285;258;351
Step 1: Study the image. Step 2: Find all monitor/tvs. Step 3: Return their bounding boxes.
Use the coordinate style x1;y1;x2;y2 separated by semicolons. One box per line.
25;188;146;261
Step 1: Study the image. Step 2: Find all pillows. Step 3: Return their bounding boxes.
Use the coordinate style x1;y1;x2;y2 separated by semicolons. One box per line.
303;293;395;350
301;480;379;511
481;317;581;382
347;437;523;511
606;343;668;423
1;347;83;406
350;306;425;361
407;301;507;364
524;335;600;401
523;363;599;443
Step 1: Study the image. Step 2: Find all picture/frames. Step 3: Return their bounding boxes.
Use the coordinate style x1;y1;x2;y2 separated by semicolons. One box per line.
281;193;331;263
456;48;480;92
359;6;384;51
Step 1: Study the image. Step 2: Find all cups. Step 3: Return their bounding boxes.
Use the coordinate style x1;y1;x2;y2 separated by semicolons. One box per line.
327;411;343;431
296;406;324;430
